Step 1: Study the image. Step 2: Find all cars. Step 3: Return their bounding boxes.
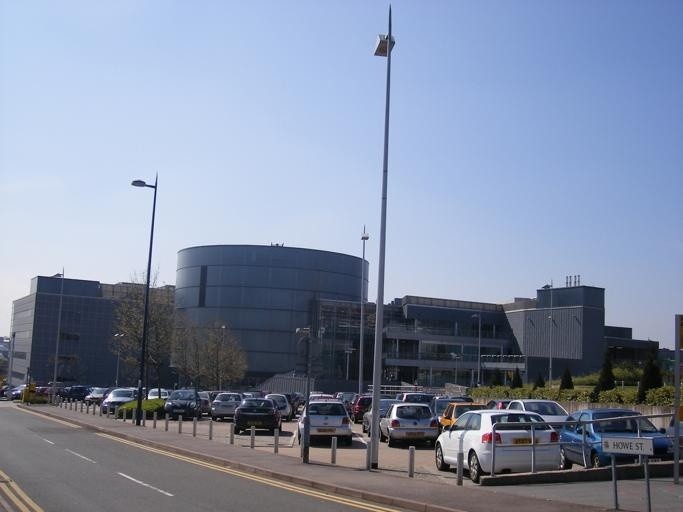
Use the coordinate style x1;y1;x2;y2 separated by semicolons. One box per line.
0;381;570;422
297;400;352;447
439;401;491;435
359;398;410;439
557;406;671;470
666;405;683;459
378;401;438;448
232;398;282;435
434;409;560;483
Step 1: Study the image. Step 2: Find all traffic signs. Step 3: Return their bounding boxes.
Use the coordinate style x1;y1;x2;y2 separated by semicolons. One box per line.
600;436;654;456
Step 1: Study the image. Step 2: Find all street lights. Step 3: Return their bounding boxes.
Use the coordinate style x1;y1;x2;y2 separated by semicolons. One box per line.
542;279;552;388
130;171;158;425
471;309;482;387
370;2;396;469
295;327;325;464
214;323;227;390
357;224;368;392
114;330;125;388
48;268;66;403
450;350;473;390
3;331;17;395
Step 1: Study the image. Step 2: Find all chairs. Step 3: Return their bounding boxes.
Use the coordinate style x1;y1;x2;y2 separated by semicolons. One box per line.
329;406;341;413
308;406;319;415
507;415;520;422
